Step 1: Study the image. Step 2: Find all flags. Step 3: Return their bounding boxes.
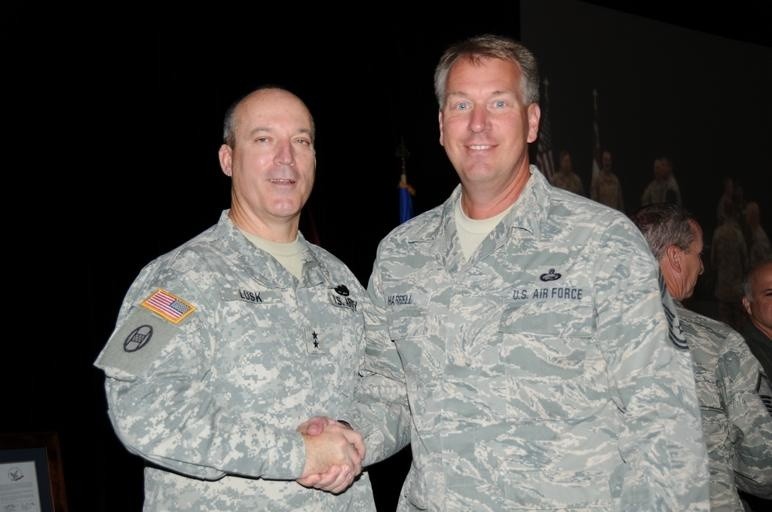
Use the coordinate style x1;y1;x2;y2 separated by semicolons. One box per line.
531;78;555;184
591;89;611;158
398;156;413;226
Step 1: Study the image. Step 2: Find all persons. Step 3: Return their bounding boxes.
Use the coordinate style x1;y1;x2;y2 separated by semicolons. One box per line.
711;178;769;330
731;240;772;383
297;34;710;512
635;201;771;511
556;149;626;213
86;89;415;511
641;153;681;208
